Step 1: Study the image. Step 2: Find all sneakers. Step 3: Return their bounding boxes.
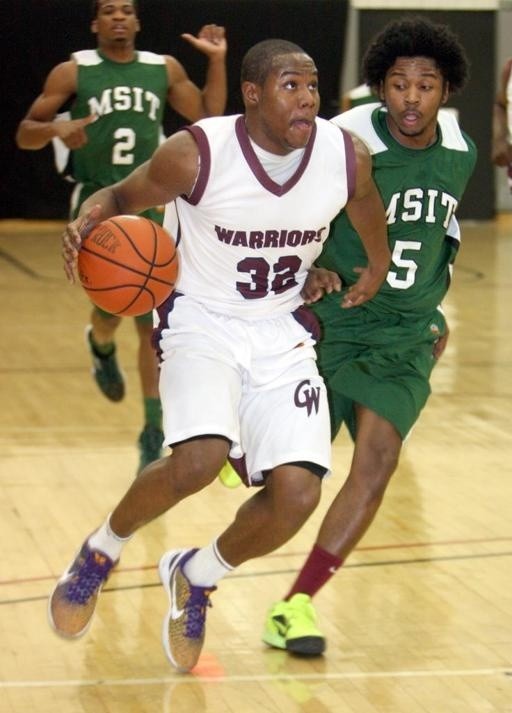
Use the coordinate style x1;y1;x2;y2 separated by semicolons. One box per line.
85;325;124;401
139;431;164;475
218;460;242;488
260;593;326;655
48;526;120;637
159;548;217;671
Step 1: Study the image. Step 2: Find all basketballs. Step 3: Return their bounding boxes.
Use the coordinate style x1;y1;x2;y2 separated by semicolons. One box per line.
77;215;179;318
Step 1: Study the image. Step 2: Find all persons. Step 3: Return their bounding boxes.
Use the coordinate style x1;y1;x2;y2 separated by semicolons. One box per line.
47;40;392;674
14;0;229;477
217;17;478;654
492;57;512;189
